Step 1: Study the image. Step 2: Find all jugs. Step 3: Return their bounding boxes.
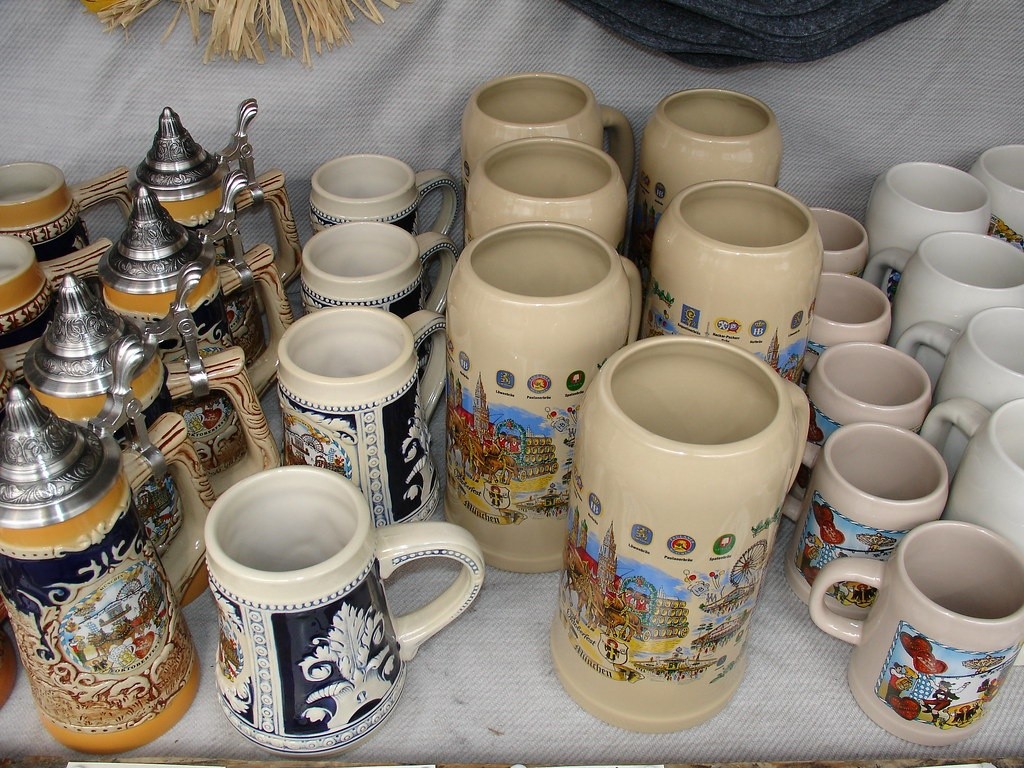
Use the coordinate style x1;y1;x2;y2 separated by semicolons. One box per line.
133;100;300;401
19;271;280;609
101;185;295;476
0;337;221;755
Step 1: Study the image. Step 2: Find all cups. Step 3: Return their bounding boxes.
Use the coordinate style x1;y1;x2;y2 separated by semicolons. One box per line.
966;143;1024;247
459;74;637;193
643;179;822;388
207;465;482;760
463;138;629;248
554;337;809;734
445;221;642;573
300;221;459;319
303;155;460;237
866;160;990;255
272;304;448;527
634;88;785;301
0;235;114;379
784;205;1024;747
0;159;134;260
